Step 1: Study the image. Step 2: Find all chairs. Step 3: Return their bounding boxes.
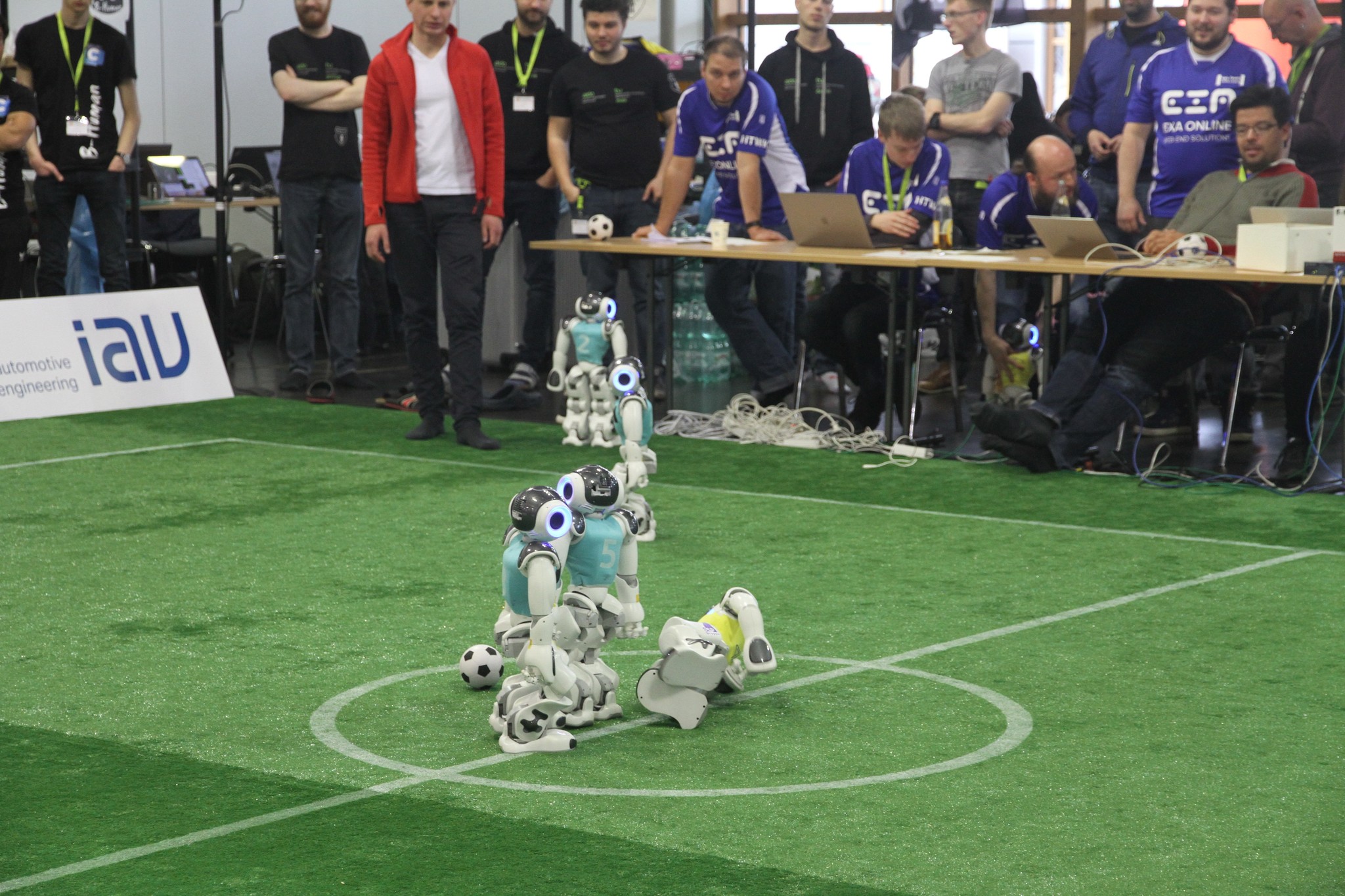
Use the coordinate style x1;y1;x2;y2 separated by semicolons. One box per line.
142;169;229;287
794;223;966;447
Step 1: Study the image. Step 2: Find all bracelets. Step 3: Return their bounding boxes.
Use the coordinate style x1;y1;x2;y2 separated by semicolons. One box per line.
746;219;762;227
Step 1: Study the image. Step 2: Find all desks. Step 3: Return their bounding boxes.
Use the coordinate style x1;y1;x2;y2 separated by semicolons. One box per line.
140;194;282;351
528;233;1337;477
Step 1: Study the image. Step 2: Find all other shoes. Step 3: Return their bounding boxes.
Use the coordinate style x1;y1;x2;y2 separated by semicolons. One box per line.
918;363;967;395
1134;409;1200;436
650;364;673;402
1223;416;1258;442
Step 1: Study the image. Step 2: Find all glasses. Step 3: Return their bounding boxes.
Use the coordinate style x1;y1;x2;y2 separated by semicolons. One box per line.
1268;12;1291;32
1234;121;1283;135
940;9;978;22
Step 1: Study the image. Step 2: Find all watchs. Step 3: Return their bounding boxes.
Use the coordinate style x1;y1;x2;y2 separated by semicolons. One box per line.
115;151;132;165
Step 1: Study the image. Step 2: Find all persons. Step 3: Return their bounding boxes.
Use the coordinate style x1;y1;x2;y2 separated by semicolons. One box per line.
360;0;506;450
267;1;372;393
440;0;576;388
547;0;682;399
0;0;141;299
632;0;1345;489
489;292;776;754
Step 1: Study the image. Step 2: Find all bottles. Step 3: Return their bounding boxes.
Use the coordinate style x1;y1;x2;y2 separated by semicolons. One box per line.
672;223;732;384
932;180;952;250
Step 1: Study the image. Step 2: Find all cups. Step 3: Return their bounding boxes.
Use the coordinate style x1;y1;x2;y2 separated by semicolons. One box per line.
711;222;729;250
147;181;163;204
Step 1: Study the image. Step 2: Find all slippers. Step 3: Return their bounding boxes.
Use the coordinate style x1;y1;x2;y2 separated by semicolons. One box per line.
383;390;426;411
481;383;538;412
507;360;540;388
440;362;458;385
307;378;337;404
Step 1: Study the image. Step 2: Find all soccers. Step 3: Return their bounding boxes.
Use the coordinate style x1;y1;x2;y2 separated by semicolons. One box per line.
586;214;614;241
1177;233;1209;258
458;642;505;688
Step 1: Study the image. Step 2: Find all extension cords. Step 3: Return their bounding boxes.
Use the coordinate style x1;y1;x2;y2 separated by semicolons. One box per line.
782;436;823;448
893;444;934;458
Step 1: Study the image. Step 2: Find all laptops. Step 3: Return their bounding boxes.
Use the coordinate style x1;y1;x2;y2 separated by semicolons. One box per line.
1027;215;1150;260
778;192;907;248
1250;205;1333;224
147;147;282;198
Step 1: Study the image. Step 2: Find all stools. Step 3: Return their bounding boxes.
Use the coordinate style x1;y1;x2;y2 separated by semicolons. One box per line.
246;253;332;357
